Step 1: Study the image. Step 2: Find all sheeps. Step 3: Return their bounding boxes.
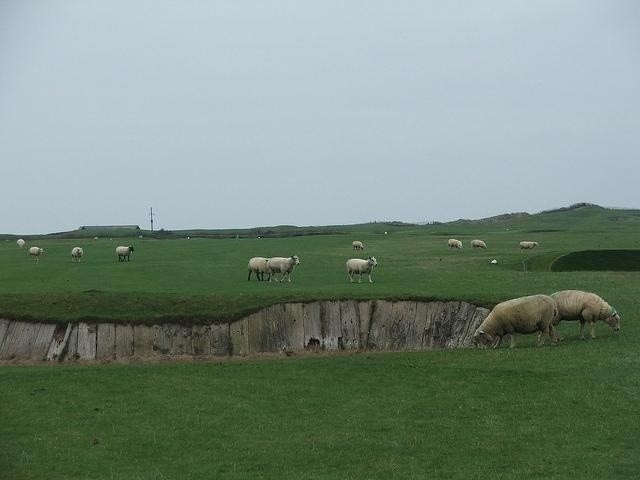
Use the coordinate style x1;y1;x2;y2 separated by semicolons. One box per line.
115;245;134;262
71;246;83;262
520;241;538;252
352;240;364;251
247;256;270;281
17;239;25;248
473;293;558;350
30;246;44;259
549;290;620;344
346;257;378;283
267;255;300;283
470;239;487;249
448;239;462;249
